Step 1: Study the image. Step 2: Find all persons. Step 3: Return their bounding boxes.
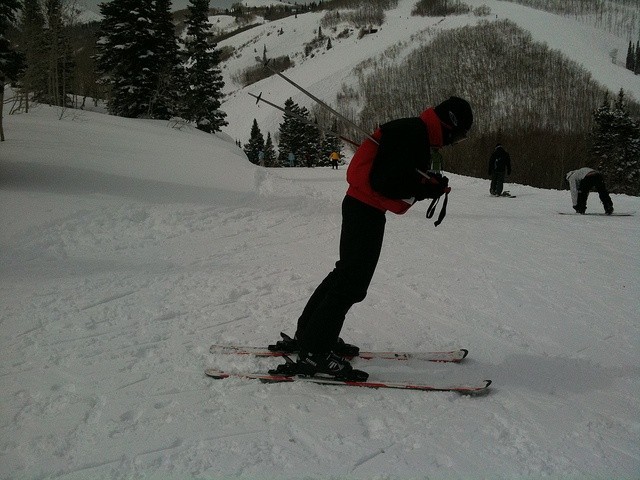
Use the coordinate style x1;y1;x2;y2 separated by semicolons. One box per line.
294;97;474;382
328;148;342;168
564;167;616;216
490;143;512;196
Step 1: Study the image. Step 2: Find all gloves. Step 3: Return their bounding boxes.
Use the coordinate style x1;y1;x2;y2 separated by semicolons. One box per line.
414;170;449;201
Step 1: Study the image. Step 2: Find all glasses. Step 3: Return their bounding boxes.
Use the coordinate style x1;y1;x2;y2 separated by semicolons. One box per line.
440;102;470;146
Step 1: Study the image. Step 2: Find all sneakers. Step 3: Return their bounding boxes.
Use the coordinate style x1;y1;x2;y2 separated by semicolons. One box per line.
297;350;352;378
294;330;346;355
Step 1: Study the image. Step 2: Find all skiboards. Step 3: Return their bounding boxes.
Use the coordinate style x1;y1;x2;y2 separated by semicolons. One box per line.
203;344;492;391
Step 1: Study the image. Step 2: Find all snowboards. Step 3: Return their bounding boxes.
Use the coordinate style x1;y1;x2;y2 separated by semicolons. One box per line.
559;212;631;216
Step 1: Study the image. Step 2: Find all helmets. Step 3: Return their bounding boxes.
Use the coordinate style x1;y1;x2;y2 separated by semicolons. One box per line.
434;96;473;147
